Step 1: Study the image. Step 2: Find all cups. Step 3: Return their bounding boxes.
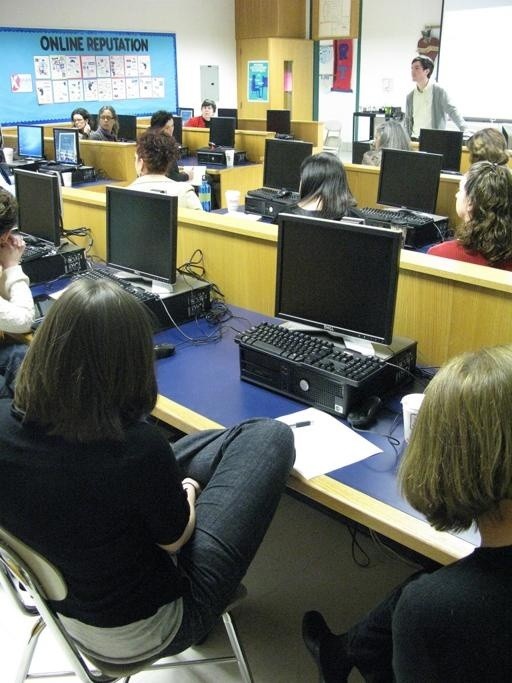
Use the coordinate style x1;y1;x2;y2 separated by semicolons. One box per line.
3;148;14;164
62;172;72;187
225;190;240;212
401;393;425;443
225;150;234;166
385;104;392;117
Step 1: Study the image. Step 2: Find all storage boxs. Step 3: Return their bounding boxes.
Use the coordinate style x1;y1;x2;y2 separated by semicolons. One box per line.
235;1;305;37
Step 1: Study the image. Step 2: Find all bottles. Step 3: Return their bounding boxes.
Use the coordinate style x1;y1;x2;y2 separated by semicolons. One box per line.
363;105;385;113
198;175;211;212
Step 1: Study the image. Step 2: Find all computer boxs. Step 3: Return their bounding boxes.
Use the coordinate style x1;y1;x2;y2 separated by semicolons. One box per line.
7;160;48;176
19;242;88;287
239;334;417;419
365;208;449;250
245;194;287;219
122;271;210;334
178;146;189;160
275;134;294;140
197;147;247;165
39;165;96;186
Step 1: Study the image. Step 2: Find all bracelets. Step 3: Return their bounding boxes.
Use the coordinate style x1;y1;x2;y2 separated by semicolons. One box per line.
181;481;198;494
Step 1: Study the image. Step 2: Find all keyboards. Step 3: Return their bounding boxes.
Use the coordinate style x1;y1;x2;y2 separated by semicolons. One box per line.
234;320;388;389
360;206;434;227
247;188;300;205
196;147;233;153
70;267;158;303
40;164;75;172
19;246;48;264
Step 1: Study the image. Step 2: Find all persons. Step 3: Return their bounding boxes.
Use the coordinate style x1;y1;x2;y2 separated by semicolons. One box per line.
150;110;174;136
300;342;511;683
125;128;207;211
465;128;509;167
254;151;364;224
426;160;512;272
405;55;469;140
1;277;296;674
70;104;125;141
0;186;35;400
185;98;218;129
361;121;415;165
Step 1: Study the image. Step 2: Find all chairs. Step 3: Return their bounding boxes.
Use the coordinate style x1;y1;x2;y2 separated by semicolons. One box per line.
0;530;256;683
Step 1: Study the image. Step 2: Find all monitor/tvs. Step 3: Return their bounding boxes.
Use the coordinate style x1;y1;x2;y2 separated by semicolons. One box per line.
13;169;61;247
263;139;314;195
267;110;290;134
376;148;444;219
53;128;80;167
17;124;44;161
218;108;238;129
210;117;235;148
419;129;463;174
179;108;194;126
274;213;402;357
106;186;178;294
87;114;98;131
116;115;137;140
172;117;182;145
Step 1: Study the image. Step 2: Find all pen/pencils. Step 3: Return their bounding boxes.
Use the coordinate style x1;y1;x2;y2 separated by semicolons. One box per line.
289;422;310;428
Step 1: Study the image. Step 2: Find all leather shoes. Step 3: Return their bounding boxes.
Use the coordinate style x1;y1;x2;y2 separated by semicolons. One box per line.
302;611;347;683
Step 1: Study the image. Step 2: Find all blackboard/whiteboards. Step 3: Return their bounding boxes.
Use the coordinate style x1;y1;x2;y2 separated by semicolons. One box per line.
436;0;512;124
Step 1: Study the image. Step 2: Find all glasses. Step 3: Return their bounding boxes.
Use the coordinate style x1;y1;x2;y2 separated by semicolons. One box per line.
101;116;116;121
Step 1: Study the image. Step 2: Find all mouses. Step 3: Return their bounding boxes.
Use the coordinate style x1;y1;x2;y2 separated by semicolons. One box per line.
347;395;382;428
153;343;175;360
278;188;292;198
48;160;58;165
